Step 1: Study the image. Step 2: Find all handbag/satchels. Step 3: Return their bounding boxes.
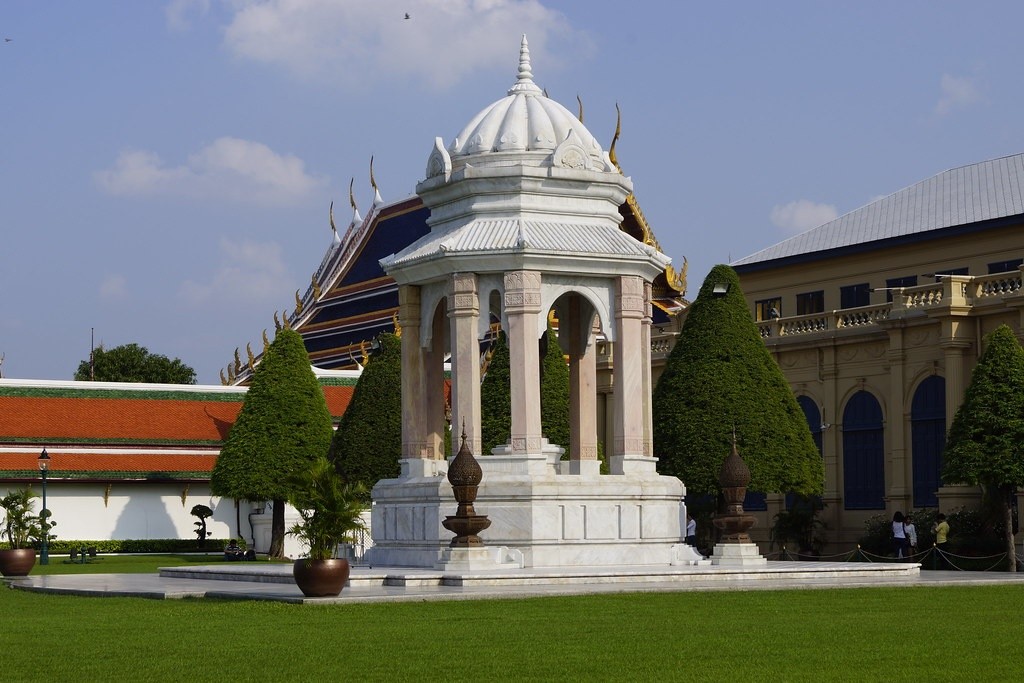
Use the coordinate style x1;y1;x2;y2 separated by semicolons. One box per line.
904;533;911;546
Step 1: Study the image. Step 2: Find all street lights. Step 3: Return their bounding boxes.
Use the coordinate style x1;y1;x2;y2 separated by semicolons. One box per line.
37;445;50;565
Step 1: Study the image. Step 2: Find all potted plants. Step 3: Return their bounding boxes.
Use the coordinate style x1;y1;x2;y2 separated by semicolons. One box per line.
0;489;57;577
281;456;374;598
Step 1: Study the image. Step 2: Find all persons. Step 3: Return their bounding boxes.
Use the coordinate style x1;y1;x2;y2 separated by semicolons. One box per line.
904;515;917;563
686;513;696;547
224;539;244;562
891;511;912;563
934;513;950;560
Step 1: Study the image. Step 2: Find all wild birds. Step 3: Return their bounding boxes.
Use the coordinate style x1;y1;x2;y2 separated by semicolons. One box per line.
404;13;410;20
